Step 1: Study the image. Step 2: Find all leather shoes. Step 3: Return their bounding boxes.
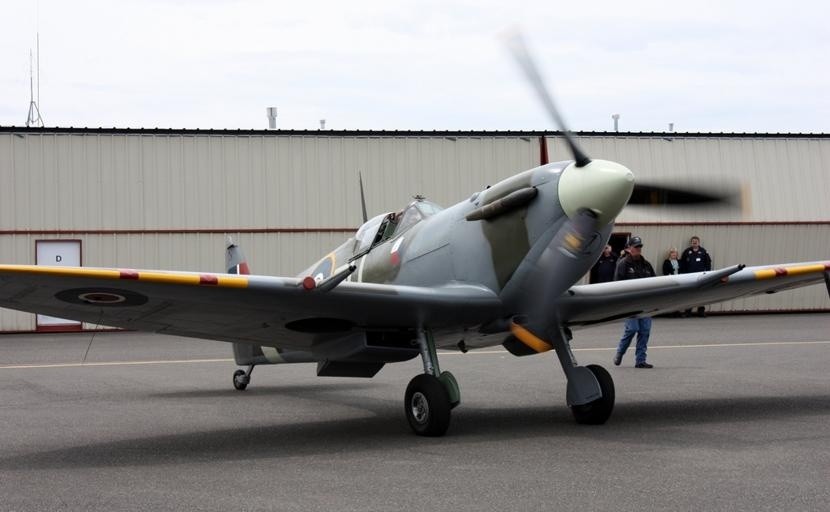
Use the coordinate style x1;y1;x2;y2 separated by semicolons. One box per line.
613;352;623;366
634;362;653;369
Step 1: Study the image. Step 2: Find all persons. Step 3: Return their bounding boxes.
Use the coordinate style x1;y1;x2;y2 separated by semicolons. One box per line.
662;247;683;319
592;242;617;284
679;235;713;320
613;236;658;370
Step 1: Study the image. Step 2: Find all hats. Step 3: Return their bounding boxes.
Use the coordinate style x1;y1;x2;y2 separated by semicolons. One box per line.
628;236;644;248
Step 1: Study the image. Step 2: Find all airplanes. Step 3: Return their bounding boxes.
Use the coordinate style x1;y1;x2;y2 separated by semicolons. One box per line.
0;30;830;434
